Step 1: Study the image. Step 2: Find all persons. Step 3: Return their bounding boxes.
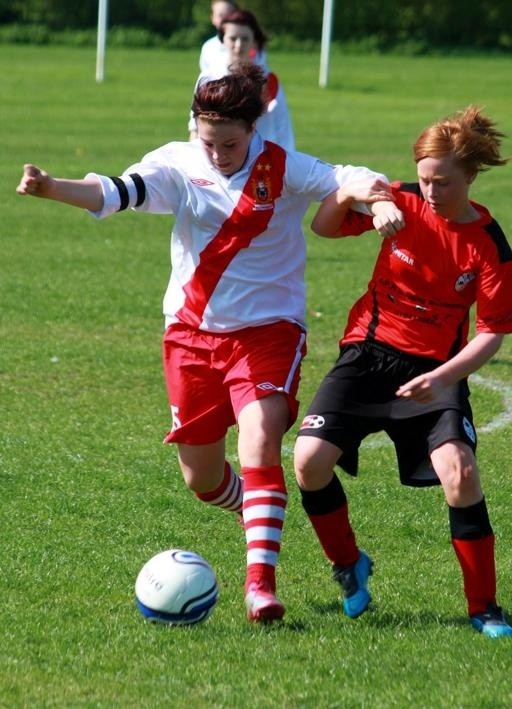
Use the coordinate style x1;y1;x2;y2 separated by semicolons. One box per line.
198;0;267;71
293;102;511;638
14;60;408;619
188;8;295;152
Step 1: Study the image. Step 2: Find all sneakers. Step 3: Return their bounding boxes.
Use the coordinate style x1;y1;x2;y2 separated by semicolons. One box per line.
332;552;373;619
246;584;284;620
469;611;512;637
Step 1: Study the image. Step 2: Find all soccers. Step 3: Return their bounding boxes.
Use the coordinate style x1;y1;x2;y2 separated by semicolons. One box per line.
133;550;218;628
